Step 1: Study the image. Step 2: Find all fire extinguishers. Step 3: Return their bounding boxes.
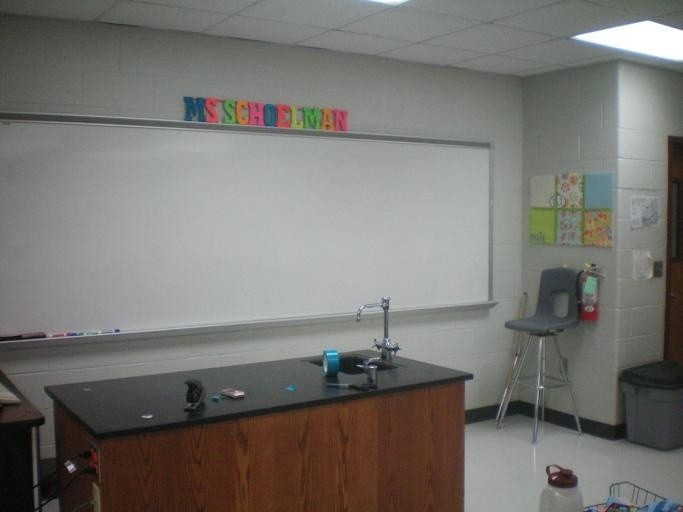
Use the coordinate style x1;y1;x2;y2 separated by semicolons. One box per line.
577;263;606;321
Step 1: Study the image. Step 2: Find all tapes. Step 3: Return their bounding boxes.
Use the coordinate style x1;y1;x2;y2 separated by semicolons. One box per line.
322;350;341;376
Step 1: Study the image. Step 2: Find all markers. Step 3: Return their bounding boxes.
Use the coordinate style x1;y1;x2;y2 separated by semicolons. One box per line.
0;336;22;341
66;332;88;336
47;332;66;338
92;328;120;334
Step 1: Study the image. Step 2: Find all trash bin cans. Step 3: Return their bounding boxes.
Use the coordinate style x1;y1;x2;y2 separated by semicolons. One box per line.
618;360;683;451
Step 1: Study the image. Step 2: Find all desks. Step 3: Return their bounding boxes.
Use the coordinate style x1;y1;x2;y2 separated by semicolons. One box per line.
45;349;474;510
1;364;44;510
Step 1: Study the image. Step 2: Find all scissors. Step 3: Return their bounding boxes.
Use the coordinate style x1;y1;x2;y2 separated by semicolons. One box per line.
325;382;378;391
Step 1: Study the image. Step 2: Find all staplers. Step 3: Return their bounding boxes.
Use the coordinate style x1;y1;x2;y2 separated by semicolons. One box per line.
183;380;205;411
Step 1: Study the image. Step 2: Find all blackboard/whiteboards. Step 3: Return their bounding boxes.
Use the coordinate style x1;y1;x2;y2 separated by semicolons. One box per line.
0;123;497;340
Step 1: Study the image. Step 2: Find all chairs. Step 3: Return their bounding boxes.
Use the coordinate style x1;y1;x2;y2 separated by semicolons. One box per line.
491;266;585;444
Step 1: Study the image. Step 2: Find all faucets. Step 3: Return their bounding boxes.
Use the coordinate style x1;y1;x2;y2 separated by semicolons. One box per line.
355;296;401;360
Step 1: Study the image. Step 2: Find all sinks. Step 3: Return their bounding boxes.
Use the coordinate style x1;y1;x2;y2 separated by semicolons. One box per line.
303;352;407;374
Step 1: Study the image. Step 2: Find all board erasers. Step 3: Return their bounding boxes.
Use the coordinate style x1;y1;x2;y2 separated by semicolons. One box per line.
19;332;47;340
